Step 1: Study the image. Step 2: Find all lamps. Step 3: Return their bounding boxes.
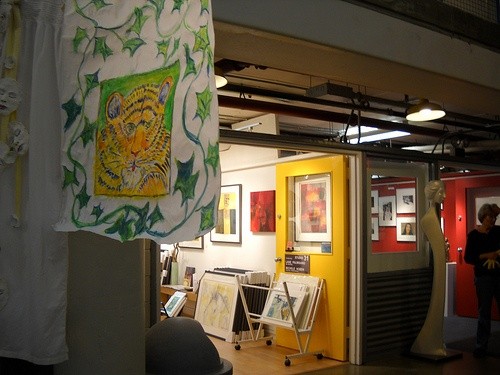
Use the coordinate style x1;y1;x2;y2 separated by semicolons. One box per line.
405;98;446;122
355;86;370;110
215;73;228;89
450;127;468;158
339;125;410;143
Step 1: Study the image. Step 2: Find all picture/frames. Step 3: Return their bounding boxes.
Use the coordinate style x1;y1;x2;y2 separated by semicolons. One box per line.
177;236;204;249
378;195;396;227
396;217;417;242
370;190;380;215
371;216;379;241
293;174;332;243
395;188;417;215
193;267;322;343
210;183;242;244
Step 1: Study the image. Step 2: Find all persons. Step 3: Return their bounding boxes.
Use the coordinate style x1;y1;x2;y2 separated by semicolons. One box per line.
463;204;500;361
425;180;447;204
